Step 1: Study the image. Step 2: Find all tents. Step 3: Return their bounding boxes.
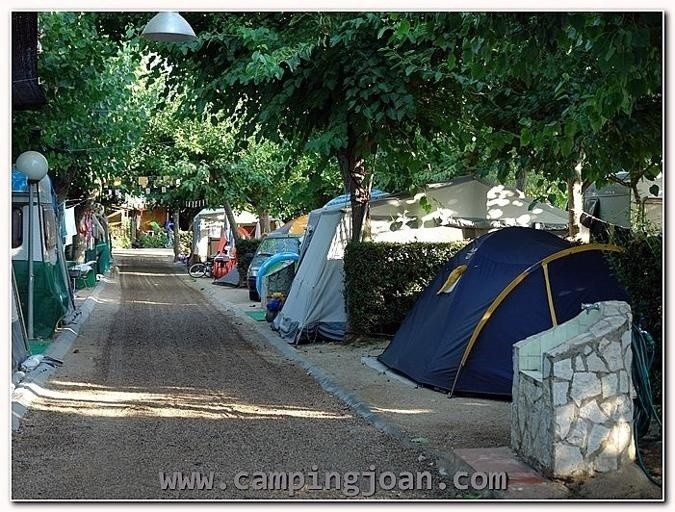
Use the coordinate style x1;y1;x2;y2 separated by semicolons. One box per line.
270;172;570;349
375;226;633;401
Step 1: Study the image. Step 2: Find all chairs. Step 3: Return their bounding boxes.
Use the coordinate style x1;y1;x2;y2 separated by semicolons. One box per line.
66;260;89;292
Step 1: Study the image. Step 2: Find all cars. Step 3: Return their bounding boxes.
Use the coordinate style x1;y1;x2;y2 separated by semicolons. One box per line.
246;233;304;300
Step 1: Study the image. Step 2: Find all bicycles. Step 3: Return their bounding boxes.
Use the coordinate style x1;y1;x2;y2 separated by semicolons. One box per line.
189;260;214;279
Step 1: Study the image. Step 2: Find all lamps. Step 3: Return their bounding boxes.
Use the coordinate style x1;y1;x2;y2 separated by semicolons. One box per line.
141;11;197;43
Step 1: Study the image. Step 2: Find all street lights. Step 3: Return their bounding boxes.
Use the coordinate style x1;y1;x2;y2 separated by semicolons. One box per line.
15;151;48;343
